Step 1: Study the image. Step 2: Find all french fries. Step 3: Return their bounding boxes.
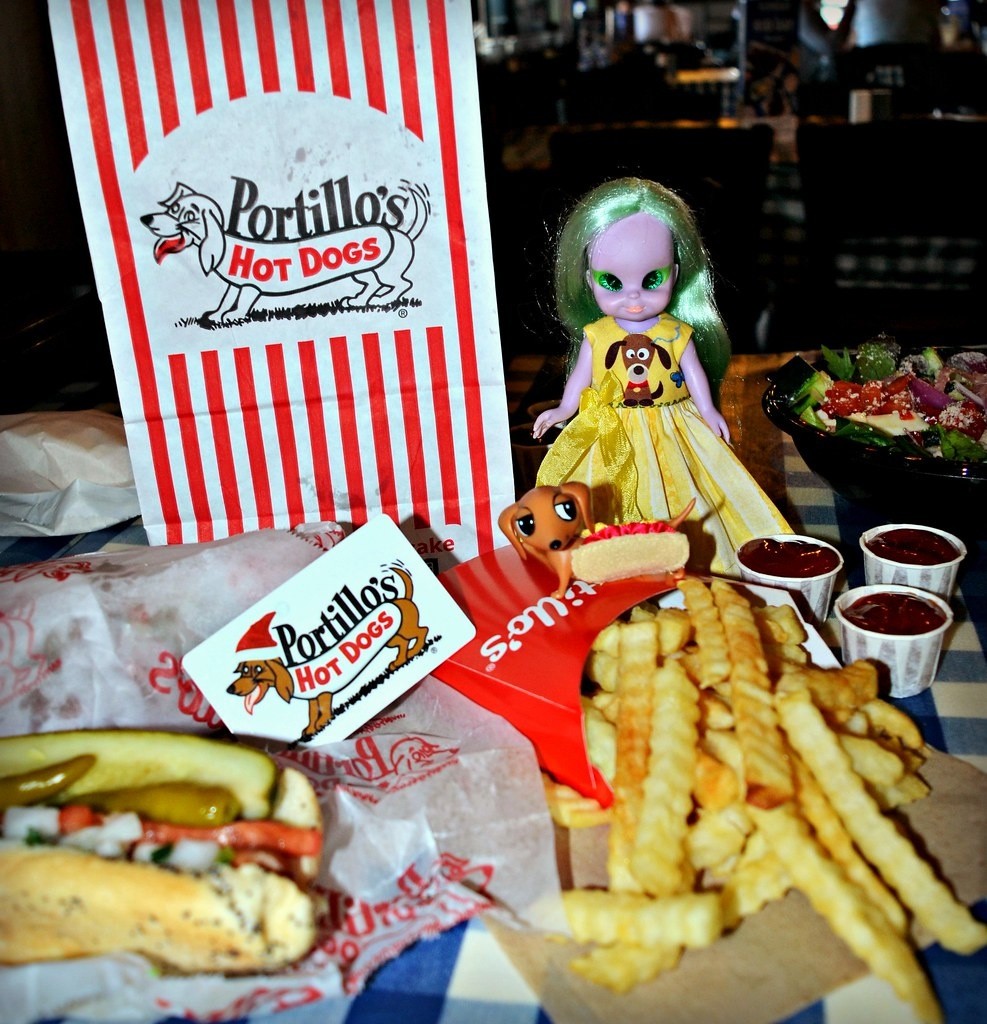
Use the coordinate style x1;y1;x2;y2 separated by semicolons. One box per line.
537;576;987;1024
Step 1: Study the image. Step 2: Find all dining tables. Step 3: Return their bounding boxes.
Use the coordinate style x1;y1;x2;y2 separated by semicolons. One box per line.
0;353;987;1024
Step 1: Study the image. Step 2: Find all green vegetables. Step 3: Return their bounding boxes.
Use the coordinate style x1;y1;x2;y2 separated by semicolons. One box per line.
802;342;987;463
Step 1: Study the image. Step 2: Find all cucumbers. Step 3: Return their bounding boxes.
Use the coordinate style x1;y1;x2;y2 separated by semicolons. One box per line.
787;370;831;415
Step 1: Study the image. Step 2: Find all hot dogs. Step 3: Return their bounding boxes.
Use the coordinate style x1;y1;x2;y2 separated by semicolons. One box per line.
1;729;324;977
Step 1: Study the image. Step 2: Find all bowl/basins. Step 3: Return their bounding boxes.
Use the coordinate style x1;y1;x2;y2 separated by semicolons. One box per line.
761;346;987;541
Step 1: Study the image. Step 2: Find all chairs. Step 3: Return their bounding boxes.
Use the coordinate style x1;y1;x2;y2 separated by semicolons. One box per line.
794;117;987;355
549;122;775;338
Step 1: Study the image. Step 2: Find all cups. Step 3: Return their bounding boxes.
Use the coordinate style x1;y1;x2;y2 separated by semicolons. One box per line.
734;534;844;629
859;523;966;605
834;584;953;699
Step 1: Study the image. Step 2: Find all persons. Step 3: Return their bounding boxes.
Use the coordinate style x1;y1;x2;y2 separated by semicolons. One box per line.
533;178;798;573
